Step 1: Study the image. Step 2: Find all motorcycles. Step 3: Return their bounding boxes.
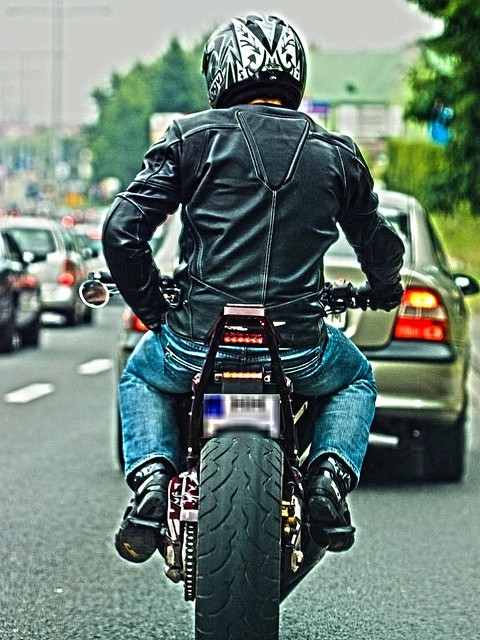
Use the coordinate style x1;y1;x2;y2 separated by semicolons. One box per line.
77;271;404;640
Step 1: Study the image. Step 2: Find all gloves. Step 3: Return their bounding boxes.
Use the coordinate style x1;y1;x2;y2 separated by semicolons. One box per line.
359;280;403;311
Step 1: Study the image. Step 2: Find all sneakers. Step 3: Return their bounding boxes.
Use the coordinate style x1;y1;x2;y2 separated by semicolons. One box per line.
306;456;355;552
114;461;171;564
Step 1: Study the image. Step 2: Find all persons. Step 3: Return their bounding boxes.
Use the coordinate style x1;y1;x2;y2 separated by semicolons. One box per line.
103;14;406;564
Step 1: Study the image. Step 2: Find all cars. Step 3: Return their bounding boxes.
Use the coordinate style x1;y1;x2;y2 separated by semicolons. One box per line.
0;233;47;353
73;222;115;297
116;190;479;484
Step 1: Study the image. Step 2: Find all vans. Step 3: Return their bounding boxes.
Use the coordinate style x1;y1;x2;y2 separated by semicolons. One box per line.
0;215;92;325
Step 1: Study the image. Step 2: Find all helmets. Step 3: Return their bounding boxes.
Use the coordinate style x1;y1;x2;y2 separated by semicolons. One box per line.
202;14;307;110
